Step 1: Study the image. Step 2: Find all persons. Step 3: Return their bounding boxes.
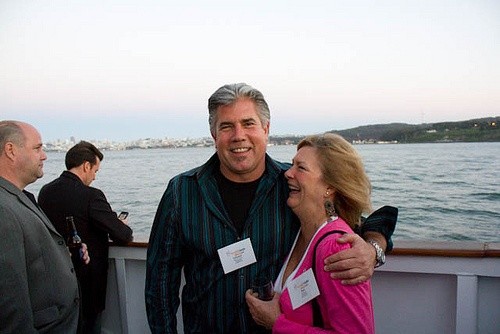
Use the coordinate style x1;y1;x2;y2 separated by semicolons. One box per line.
0;120;90;334
244;132;375;334
37;140;133;334
144;82;399;334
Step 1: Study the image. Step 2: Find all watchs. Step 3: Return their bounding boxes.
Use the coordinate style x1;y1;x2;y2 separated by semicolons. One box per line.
368;239;386;269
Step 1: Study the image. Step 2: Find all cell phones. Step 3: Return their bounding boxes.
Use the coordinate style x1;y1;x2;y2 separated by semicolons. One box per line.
118;212;128;221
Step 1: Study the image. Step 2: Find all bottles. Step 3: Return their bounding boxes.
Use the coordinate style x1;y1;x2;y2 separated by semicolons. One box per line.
65;216;86;269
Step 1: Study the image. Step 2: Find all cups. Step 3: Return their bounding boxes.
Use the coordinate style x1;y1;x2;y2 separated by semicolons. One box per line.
250;279;276;303
